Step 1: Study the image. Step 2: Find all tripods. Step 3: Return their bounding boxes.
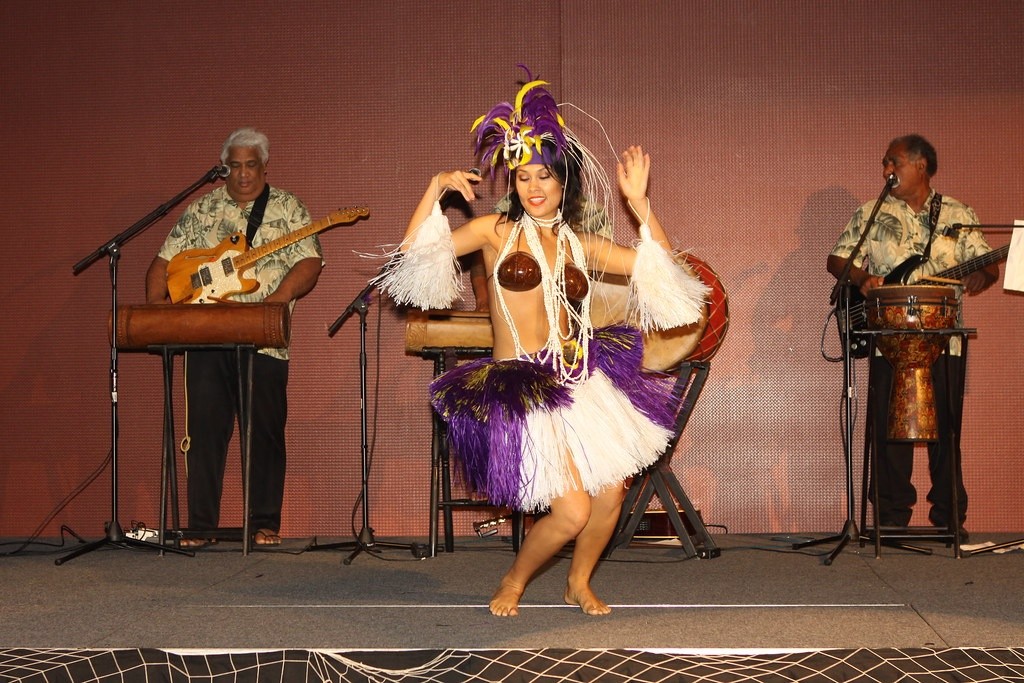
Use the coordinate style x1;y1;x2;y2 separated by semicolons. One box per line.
305;252;445;565
789;179;933;566
53;165;219;567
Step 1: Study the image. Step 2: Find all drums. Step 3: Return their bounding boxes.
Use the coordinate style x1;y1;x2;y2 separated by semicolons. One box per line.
864;284;961;441
588;248;730;380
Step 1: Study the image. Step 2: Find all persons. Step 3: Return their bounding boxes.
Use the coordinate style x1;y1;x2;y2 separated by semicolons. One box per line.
145;125;327;551
826;132;1000;545
398;131;677;617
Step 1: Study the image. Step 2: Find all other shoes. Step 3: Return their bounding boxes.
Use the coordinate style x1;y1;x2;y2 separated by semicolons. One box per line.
935;523;970;543
870;523;911;545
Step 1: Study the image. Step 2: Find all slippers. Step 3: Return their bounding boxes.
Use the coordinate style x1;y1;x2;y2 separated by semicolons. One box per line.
252;529;280;546
179;537;219;549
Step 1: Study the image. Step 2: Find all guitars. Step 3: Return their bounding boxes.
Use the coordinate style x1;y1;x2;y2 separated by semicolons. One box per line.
827;240;1010;361
165;202;371;304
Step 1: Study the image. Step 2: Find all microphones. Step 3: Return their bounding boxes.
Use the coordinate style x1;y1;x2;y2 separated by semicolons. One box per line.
887;173;900;188
215;165;231;177
941;227;960;238
